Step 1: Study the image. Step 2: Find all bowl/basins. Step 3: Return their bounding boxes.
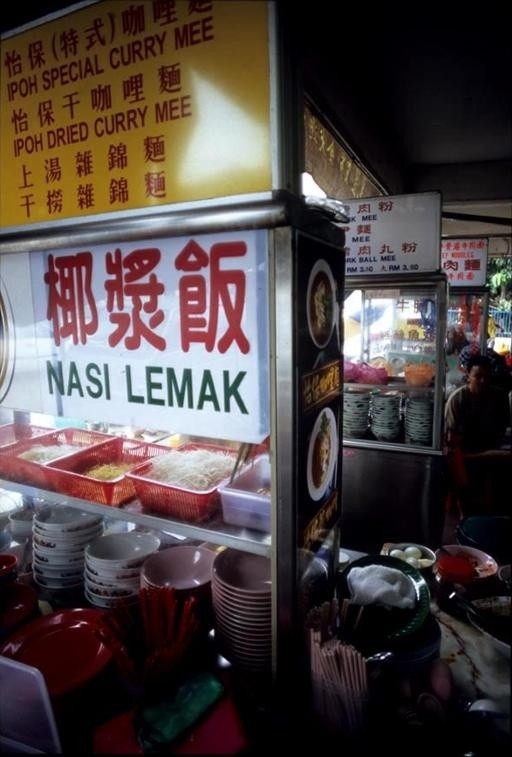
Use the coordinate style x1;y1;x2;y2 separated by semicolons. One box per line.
140;545;217;659
337;543;512;658
337;391;433;446
1;506;104;604
210;547;271;667
82;531;162;610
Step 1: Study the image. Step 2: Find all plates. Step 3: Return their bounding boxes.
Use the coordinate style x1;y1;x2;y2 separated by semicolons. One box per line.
2;607;123;702
307;407;338;502
307;258;338;350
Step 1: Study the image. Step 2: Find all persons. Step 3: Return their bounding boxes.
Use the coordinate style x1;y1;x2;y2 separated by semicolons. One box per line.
444;356;511;450
